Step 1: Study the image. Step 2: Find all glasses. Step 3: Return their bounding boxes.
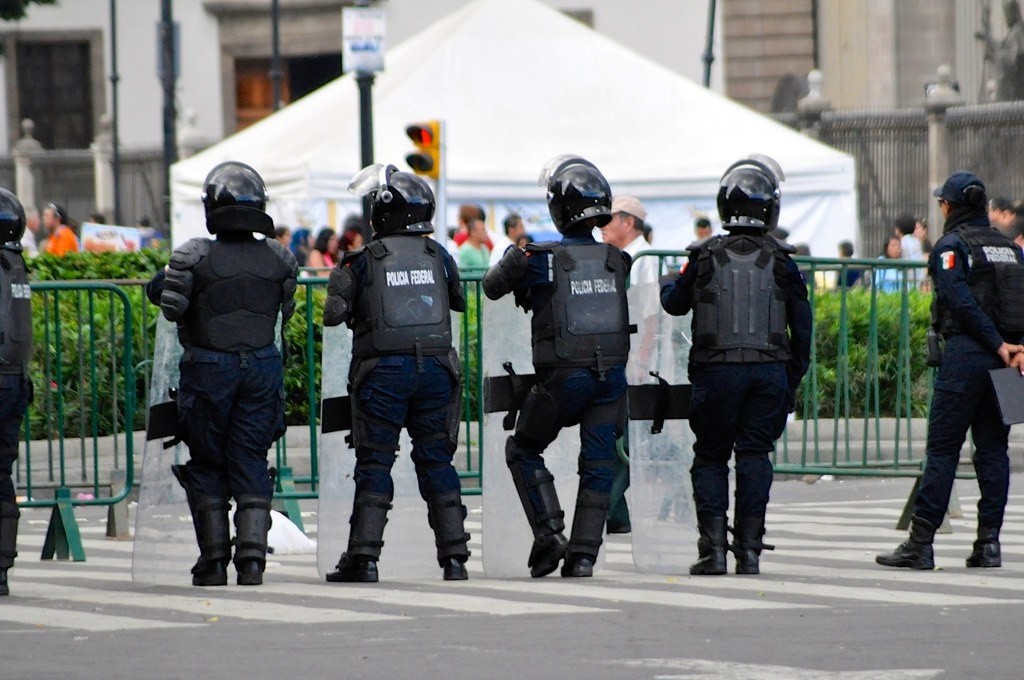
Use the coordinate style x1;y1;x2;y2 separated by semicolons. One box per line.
938;196;946;207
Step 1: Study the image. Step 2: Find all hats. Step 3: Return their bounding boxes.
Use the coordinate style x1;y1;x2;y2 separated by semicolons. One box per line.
610;195;648;222
933;172;986;205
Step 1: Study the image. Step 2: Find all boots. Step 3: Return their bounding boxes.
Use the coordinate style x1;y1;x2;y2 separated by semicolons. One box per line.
966;527;1001;567
876;513;936;569
735;517;764;574
690;517;727;575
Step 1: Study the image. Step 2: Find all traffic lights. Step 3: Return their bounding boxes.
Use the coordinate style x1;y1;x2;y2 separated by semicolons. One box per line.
405;121;440;178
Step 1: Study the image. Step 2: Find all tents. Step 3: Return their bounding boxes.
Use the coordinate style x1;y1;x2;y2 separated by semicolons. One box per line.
168;0;861;290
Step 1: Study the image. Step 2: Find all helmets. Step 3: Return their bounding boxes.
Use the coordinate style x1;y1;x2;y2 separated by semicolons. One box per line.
201;161;274;239
716;168;781;234
372;172;436;235
0;187;26;254
546;166;612;233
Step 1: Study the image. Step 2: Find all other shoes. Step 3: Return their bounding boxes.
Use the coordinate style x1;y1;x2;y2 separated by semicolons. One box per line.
444;558;468;580
326;555;378;582
561;553;592;577
191;559;227;586
530;533;569;578
235;557;265;585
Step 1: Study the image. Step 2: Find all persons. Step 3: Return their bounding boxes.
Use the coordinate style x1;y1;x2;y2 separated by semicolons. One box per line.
446;206;534;276
483;155;633;578
874;212;932;297
40;201;79;258
659;158;814;575
873;169;1024;570
91;213;105;224
643;224;653;244
794;244;812;285
0;187;34;597
837;244;862;290
680;219;712;273
141;218;163;249
20;203;41;259
988;197;1024;251
147;162;300;585
273;215;363;276
323;163;471;583
600;195;676;383
